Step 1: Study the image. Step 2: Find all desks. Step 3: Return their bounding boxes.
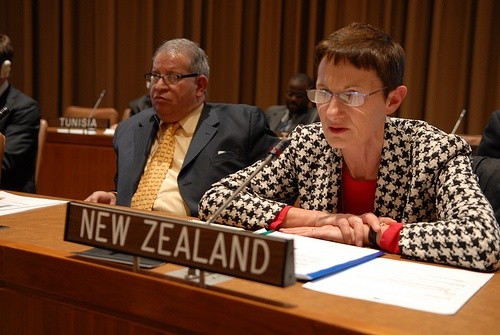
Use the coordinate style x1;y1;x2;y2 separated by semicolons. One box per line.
38;125;483;205
0;190;500;335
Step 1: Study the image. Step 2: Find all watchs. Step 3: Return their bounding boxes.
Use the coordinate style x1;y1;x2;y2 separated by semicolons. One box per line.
368;221;386;247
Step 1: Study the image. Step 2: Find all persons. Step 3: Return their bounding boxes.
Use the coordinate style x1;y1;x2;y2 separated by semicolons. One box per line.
129;93;153;117
83;39;299;218
475;110;500;159
0;34;41;194
265;74;320;138
198;23;500;273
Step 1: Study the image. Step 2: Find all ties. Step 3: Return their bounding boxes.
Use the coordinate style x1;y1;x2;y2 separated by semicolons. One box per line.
129;122;181;212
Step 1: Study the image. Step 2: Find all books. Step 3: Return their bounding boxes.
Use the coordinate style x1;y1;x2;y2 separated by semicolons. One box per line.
184;220;494;315
0;191;70;216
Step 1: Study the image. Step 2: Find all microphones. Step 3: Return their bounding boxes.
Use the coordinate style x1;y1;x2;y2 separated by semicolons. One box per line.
87;89;106;131
451;110;466;134
206;138;291;223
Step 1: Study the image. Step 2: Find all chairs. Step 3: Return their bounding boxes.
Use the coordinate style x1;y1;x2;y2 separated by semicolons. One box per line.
65;106;118;128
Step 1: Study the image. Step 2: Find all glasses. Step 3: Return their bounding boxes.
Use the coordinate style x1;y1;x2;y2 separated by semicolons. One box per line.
304;84;388;108
144;71;198;87
